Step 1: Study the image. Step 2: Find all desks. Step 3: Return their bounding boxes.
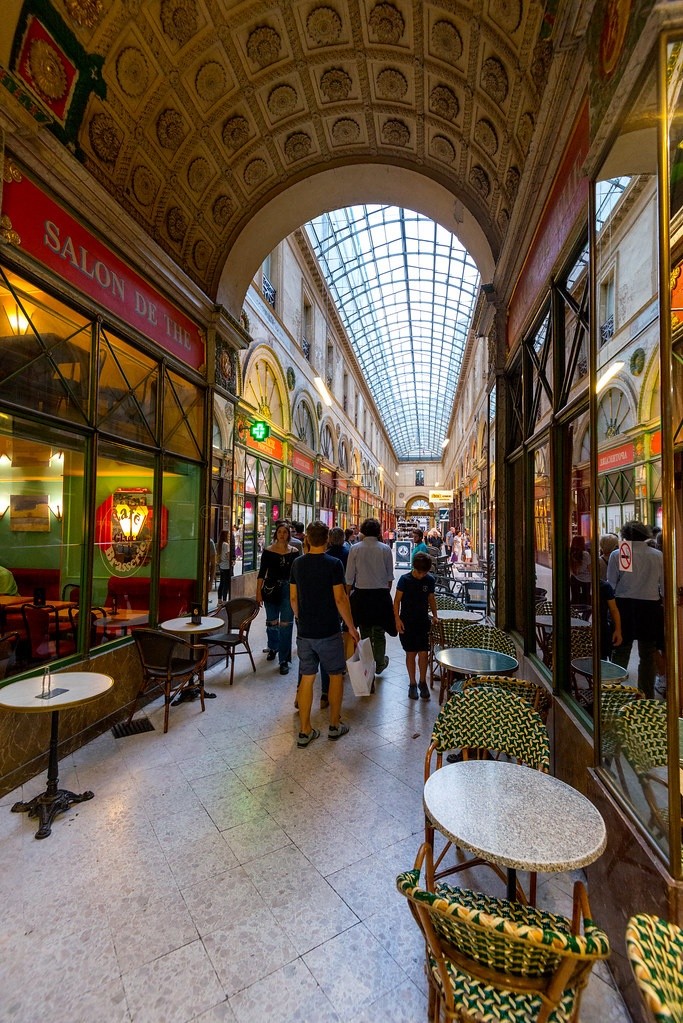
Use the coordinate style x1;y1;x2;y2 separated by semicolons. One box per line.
422;760;608;897
0;670;116;840
454;575;487;604
162;616;224;698
571;655;629;710
434;649;520;702
0;596;149;628
456;566;484;577
536;612;590;666
0;584;127;682
455;561;478;577
428;609;483;622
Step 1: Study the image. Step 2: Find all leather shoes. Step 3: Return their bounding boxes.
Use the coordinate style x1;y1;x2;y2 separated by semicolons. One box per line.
267;648;278;660
279;661;289;674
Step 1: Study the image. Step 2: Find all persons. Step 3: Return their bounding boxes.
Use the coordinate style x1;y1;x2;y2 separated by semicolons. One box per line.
0;566;19;633
329;517;400;675
256;519;309;675
294;536;330;708
290;522;361;747
393;553;439;700
571;521;666;696
412;527;472;567
210;526;241;605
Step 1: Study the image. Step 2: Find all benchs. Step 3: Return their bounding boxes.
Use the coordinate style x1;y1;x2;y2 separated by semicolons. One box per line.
5;567;61;602
103;576;199;620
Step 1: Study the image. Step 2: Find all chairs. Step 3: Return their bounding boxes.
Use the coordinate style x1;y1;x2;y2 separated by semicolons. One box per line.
601;684;644;808
202;597;259;685
613;698;683;865
536;601;583;647
130;626;207;735
548;627;596;670
397;550;683;1023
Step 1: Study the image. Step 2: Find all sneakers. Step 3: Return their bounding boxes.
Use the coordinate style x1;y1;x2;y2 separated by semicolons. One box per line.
327;721;348;740
297;728;319;748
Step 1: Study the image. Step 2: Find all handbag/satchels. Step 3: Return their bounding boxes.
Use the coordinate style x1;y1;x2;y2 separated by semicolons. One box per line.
451;552;458;562
345;637;376;696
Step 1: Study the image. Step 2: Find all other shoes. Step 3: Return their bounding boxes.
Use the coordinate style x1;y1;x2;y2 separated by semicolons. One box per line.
376;656;388;674
319;694;329;708
409;682;418;699
417;680;430;699
295;691;300;709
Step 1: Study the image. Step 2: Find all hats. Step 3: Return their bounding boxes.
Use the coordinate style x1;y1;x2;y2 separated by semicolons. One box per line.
412;529;422;537
